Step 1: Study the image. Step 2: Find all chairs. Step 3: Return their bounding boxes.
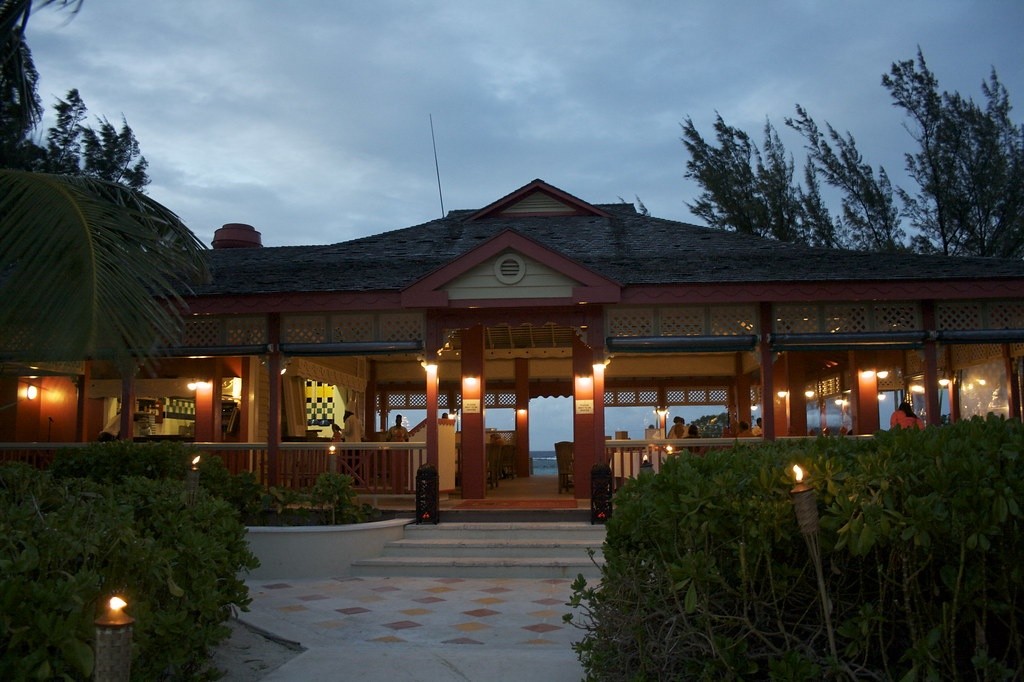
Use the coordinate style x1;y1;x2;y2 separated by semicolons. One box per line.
484;443;516;487
554;441;574;493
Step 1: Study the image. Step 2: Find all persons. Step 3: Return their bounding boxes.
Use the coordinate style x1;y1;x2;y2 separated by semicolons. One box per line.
890;403;925;431
341;410;363;442
679;424;702;453
97;412;156;441
332;424;342;442
752;418;764;437
668;416;689;452
386;414;409;442
736;421;754;437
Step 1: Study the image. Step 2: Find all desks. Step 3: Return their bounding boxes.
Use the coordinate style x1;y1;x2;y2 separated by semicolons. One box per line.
608;452;686;493
